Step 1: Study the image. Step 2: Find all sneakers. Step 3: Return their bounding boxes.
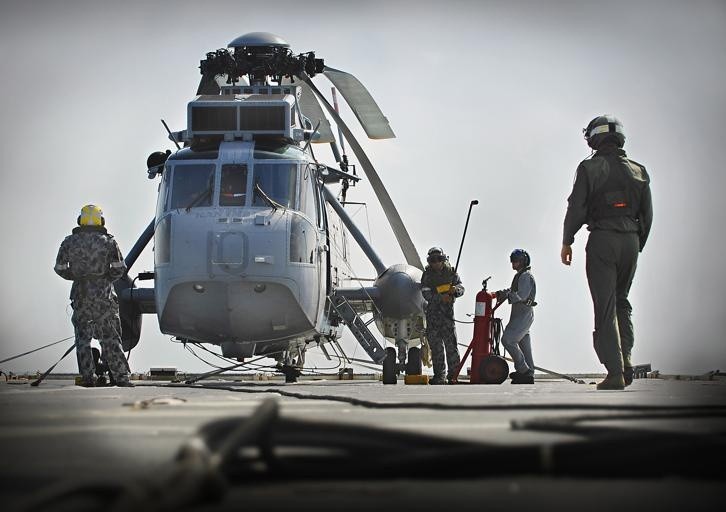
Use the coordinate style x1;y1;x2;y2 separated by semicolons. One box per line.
508;368;534;383
429;377;458;385
596;374;625;390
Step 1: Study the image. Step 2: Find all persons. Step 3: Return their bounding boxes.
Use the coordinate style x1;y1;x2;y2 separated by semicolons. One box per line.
421;247;465;384
561;114;653;390
497;249;536;384
53;204;135;387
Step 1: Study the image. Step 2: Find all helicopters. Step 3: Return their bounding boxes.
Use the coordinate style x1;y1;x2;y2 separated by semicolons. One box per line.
112;29;432;386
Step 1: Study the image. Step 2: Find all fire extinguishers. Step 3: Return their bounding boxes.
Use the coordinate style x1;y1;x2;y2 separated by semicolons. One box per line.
470;276;497;385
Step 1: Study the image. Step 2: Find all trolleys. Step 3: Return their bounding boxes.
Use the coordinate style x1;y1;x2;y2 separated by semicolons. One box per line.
452;294;513;386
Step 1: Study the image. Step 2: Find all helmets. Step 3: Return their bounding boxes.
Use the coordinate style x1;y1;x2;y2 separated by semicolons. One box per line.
80;205;102;226
583;116;625;149
511;250;530;266
427;247;445;263
